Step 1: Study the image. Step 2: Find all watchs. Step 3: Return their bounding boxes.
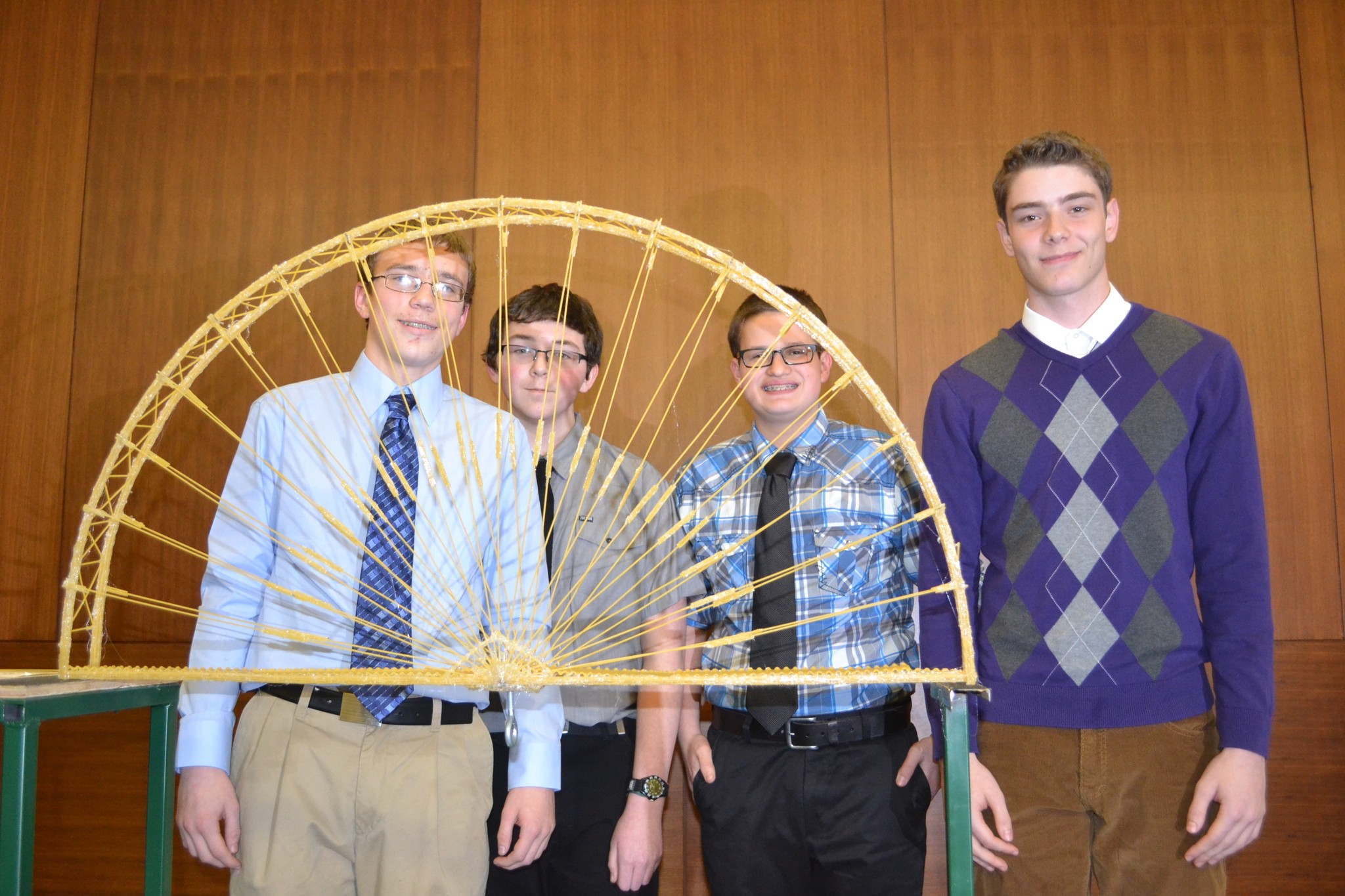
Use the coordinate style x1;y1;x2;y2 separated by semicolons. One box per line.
629;775;669;801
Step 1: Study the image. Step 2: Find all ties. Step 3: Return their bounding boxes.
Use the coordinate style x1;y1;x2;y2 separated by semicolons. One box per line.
744;453;800;734
352;394;417;723
533;456;555;580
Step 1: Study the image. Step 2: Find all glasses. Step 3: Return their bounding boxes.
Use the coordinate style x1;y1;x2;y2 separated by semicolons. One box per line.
737;343;819;368
369;271;466;304
495;343;587;370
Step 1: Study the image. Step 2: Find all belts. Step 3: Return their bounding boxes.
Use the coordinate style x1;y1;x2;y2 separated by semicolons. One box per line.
711;700;911;749
560;715;639;736
259;678;478;728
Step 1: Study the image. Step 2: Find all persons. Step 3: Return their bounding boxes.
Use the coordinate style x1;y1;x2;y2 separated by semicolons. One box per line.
174;214;565;896
479;281;707;895
671;285;943;896
918;128;1272;896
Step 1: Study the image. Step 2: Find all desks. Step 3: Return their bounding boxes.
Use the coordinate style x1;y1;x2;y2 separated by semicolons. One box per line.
0;669;181;896
